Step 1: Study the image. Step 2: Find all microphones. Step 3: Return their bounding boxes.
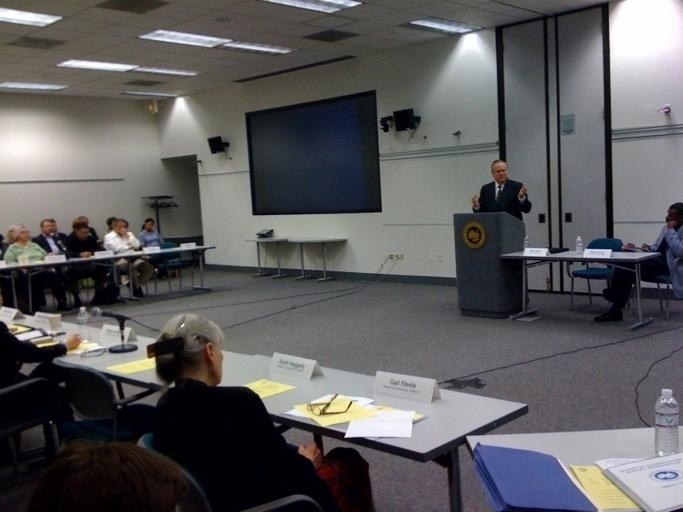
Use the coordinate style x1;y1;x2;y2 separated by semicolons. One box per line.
91;306;132;320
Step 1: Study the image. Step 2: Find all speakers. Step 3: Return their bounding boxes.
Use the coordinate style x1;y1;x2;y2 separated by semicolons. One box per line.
393;109;415;131
208;136;225;154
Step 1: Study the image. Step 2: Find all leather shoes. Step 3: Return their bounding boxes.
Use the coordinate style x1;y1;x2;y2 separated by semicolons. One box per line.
601;287;622;301
594;309;625;324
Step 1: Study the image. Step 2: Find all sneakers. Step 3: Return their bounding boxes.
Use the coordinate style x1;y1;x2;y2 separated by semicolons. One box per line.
128;281;146;298
59;296;82;312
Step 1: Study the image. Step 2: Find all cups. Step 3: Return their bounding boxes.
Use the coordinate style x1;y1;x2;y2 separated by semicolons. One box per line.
17;256;28;265
0;261;6;267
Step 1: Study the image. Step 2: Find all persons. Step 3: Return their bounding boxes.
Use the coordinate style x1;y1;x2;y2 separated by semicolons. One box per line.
150;314;374;512
24;440;212;512
0;295;81;460
471;159;532;220
594;203;683;322
0;217;164;311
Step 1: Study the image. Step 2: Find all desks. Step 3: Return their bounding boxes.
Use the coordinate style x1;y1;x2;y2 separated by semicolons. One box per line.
0;308;529;512
245;238;346;282
455;423;683;512
499;246;663;331
0;241;217;316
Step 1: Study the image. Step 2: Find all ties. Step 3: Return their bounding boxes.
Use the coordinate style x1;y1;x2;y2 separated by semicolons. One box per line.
497;184;503;203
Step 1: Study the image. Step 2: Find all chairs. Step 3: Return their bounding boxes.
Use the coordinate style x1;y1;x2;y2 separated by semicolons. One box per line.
640;275;674;322
567;236;624;311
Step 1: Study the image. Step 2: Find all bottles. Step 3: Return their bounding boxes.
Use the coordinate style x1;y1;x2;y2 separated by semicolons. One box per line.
653;389;678;457
77;307;89;341
575;236;583;255
